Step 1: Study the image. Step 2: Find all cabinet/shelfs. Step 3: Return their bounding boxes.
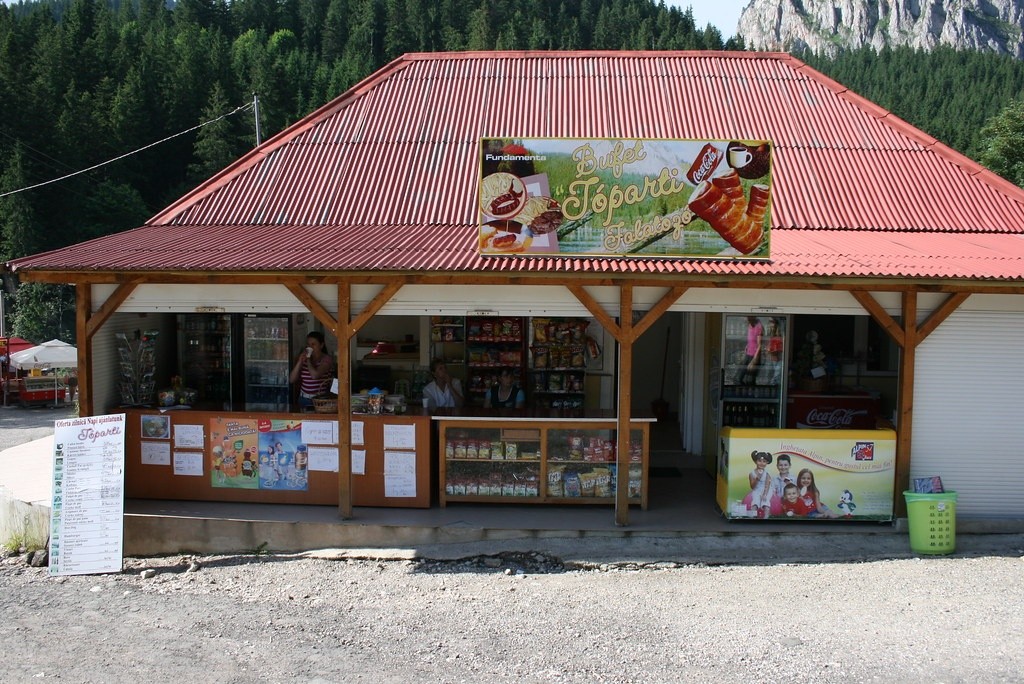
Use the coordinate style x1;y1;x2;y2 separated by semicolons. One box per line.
430;317;588;418
436;421;650;511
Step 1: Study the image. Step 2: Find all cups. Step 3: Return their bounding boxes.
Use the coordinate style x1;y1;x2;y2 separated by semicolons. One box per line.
729;147;752;167
306;348;313;358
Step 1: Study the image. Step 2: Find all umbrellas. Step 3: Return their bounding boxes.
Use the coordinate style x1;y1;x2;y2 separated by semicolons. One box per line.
7;338;78;406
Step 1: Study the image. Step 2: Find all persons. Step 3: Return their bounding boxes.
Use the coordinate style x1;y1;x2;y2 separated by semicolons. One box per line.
733;317;782;385
482;365;526;409
743;451;838;518
291;330;335;412
422;357;464;407
267;442;289;480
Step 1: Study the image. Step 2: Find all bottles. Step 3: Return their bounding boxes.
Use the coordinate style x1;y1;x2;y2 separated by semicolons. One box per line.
185;316;230;412
244;319;289;413
723;316;783;427
295;446;308;487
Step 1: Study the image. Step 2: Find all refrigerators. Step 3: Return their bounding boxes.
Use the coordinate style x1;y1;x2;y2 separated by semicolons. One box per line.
180;313;237;412
703;313;791;478
715;427;896;522
238;313;309;413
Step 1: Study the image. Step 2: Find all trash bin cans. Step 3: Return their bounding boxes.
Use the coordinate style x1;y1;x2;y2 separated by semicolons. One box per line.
902;488;957;555
